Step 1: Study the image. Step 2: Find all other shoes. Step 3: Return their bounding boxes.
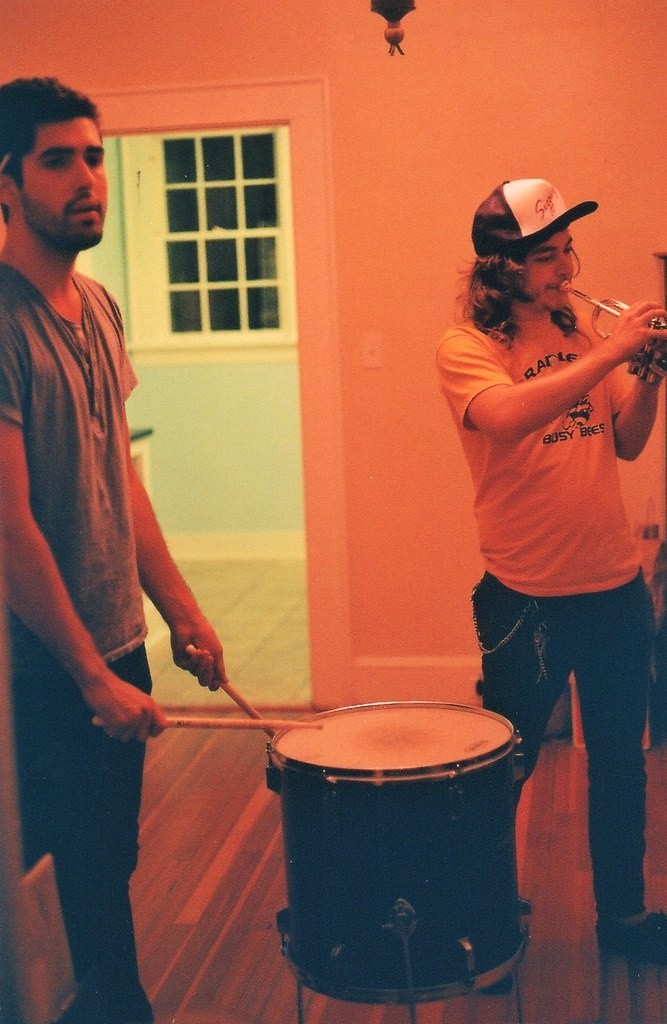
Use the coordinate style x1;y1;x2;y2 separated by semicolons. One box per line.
518;897;532;913
594;908;667;965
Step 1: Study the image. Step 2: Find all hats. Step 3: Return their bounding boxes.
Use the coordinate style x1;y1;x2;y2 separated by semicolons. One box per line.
471;179;598;258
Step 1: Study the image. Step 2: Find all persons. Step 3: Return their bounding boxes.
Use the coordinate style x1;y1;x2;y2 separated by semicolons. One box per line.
0;77;227;1024
437;178;667;963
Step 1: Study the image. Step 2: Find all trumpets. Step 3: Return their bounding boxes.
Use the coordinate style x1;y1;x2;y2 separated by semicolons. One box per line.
557;279;667;384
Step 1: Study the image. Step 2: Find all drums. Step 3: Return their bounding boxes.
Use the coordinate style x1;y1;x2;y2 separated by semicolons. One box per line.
264;699;534;1006
